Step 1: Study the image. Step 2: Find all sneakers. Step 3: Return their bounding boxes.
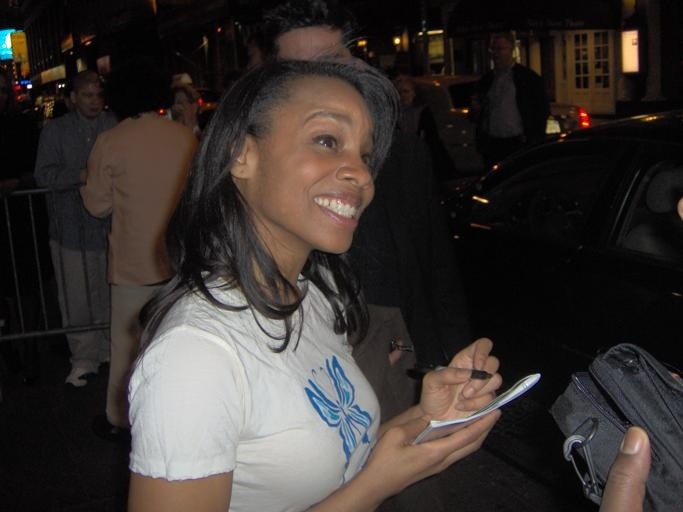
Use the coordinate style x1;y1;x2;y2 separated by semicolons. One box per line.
63;365;99;388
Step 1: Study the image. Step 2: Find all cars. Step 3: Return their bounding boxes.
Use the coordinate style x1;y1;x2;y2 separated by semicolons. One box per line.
439;110;683;377
413;75;479;159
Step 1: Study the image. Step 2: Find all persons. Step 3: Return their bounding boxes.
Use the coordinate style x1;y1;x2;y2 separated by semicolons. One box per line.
392;74;458;181
29;54;241;439
598;426;652;512
467;32;550;173
126;57;501;512
252;6;478;510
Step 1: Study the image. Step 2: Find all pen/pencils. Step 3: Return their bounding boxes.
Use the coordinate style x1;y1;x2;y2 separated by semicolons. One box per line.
416;359;492;380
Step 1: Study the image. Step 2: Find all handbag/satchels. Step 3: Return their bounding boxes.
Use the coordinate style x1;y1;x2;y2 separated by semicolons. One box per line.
548;343;681;511
